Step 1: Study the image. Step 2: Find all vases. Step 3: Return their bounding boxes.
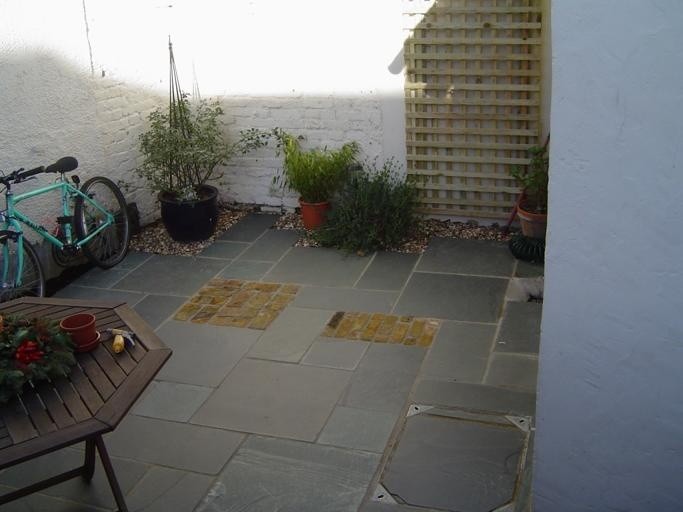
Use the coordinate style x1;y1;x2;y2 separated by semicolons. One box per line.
58;310;95;346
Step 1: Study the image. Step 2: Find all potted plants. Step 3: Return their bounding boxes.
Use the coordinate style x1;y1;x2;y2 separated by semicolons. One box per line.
116;88;272;242
510;145;549;239
273;125;359;227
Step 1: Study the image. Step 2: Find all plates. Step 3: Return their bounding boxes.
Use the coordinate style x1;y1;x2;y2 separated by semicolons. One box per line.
75;331;101;353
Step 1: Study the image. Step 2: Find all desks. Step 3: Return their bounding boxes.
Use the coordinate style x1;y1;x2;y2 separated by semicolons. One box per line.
0;296;175;512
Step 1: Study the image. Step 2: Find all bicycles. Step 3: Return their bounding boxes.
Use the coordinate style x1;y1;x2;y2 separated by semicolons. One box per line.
0;153;136;307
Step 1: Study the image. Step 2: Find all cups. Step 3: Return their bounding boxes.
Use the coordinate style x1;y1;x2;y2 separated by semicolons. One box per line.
59;313;96;345
